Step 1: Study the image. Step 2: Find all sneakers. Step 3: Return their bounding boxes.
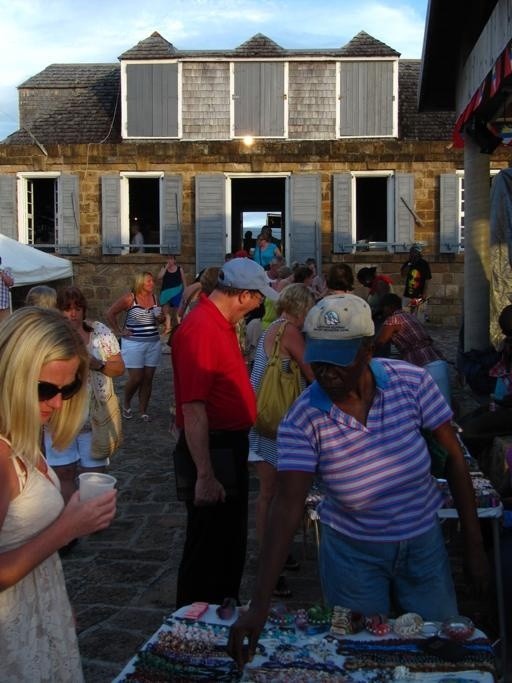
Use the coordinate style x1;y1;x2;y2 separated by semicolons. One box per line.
285;555;300;571
273;571;296;596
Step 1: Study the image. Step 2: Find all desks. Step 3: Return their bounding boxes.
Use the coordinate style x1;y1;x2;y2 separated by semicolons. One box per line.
111;602;503;683
306;421;512;680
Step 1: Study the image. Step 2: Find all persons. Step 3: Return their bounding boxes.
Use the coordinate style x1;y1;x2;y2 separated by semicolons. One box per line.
0;223;511;682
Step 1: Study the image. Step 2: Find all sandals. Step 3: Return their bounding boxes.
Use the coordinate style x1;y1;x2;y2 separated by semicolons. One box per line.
160;327;173;337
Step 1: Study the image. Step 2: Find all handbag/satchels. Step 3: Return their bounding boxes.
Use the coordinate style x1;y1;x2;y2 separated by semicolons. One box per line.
167;324;177;346
252;357;301;437
90;394;124;460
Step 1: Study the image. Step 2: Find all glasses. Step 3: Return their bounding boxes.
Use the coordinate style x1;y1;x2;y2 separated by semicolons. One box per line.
37;375;82;402
254;294;265;304
259;238;265;241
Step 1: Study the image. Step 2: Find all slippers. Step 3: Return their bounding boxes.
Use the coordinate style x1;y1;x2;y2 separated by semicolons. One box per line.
123;404;133;419
141;415;152;422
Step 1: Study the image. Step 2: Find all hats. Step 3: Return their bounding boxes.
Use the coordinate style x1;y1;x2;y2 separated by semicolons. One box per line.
303;293;375;368
356;266;376;286
218;258;280;302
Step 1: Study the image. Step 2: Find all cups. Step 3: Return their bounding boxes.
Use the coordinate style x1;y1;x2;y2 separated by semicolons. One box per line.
153;307;161;317
78;472;117;504
